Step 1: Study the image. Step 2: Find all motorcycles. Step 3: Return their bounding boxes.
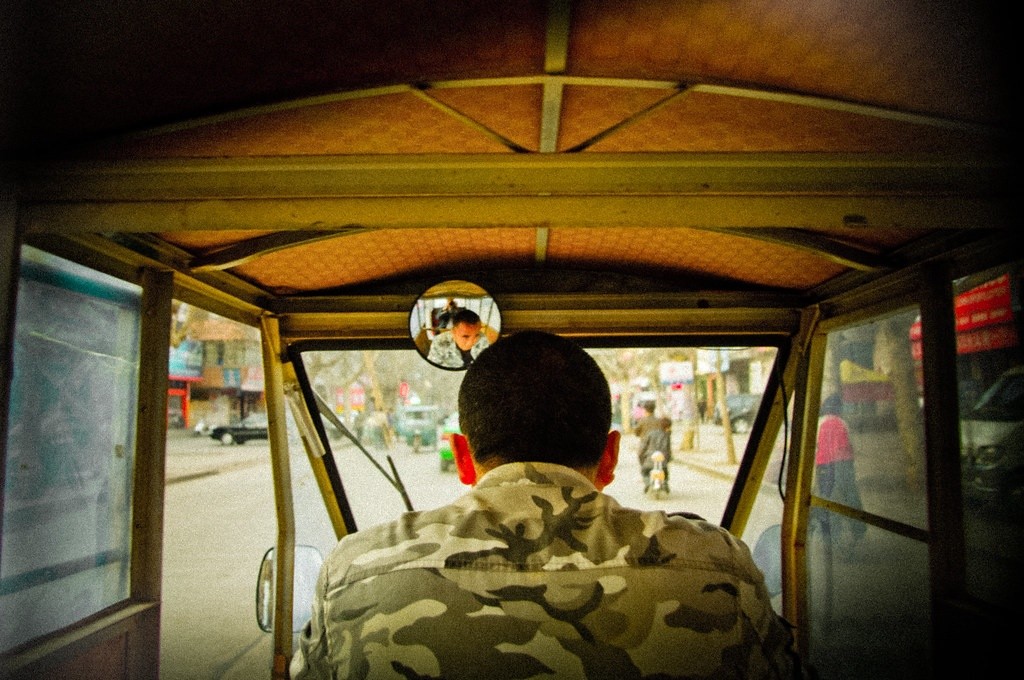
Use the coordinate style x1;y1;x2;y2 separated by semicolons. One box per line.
397;405;439;454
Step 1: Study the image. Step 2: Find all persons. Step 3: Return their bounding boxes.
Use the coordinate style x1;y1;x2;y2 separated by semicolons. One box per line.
813;394;868;539
426;302;490;370
289;329;814;680
351;397;401;448
613;383;706;493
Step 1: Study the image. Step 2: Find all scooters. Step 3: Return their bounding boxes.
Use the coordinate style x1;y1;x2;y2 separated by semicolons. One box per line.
637;434;667;501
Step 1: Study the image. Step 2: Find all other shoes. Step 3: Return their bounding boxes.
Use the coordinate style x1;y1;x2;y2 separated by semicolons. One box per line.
664;483;670;493
644;484;649;493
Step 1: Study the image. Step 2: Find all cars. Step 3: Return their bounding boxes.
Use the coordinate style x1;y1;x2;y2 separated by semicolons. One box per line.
208;412;268;446
713;392;764;436
959;366;1024;514
